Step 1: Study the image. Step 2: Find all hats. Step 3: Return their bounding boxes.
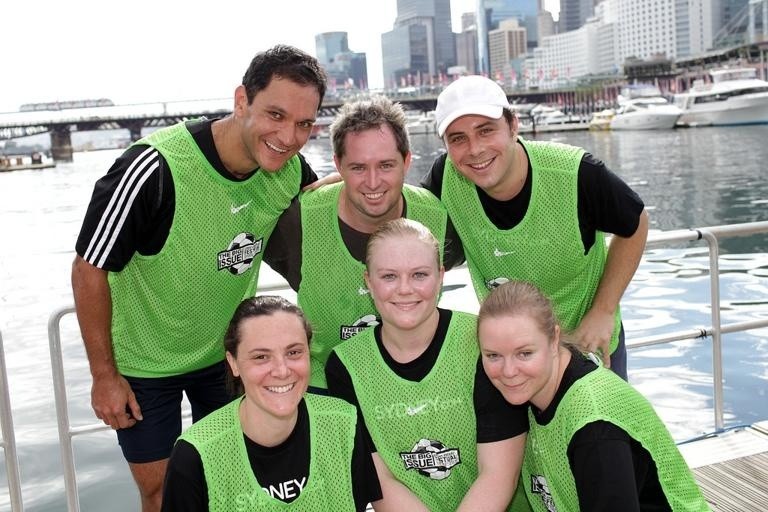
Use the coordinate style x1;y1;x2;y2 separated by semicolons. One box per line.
435;74;513;139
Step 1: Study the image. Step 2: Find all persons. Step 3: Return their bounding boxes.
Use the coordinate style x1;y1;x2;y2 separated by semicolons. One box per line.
260;98;466;397
324;208;532;511
300;71;649;381
69;43;328;511
158;295;383;510
474;279;710;511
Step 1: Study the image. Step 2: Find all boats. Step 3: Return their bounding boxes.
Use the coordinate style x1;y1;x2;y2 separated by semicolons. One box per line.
0;152;56;171
518;102;591;133
588;76;684;131
673;66;768;128
